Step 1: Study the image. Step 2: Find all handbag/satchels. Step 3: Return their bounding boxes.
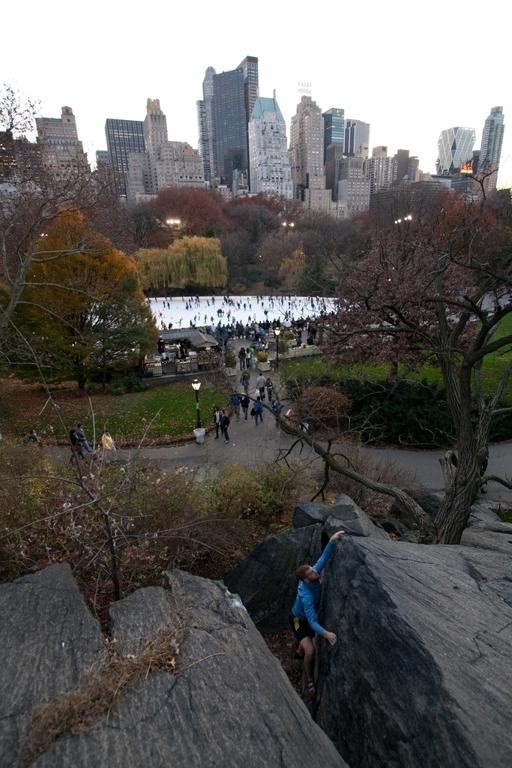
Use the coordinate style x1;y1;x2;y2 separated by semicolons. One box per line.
251;408;256;416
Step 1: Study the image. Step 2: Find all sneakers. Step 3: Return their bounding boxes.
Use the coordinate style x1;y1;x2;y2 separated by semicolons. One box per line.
308;681;316;694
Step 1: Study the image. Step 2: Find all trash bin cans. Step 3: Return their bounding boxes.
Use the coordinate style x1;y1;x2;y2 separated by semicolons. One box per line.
193;427;206;445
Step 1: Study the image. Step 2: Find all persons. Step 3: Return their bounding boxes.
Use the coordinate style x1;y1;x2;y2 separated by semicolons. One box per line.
76;423;92;460
479;458;488;494
287;530;346;700
143;293;335;445
28;429;42;444
69;429;88;465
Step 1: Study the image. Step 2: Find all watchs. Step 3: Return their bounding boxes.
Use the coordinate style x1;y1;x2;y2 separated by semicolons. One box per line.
101;430;117;466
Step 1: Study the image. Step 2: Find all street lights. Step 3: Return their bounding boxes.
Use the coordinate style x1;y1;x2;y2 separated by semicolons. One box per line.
191;378;202;428
275;327;280;367
